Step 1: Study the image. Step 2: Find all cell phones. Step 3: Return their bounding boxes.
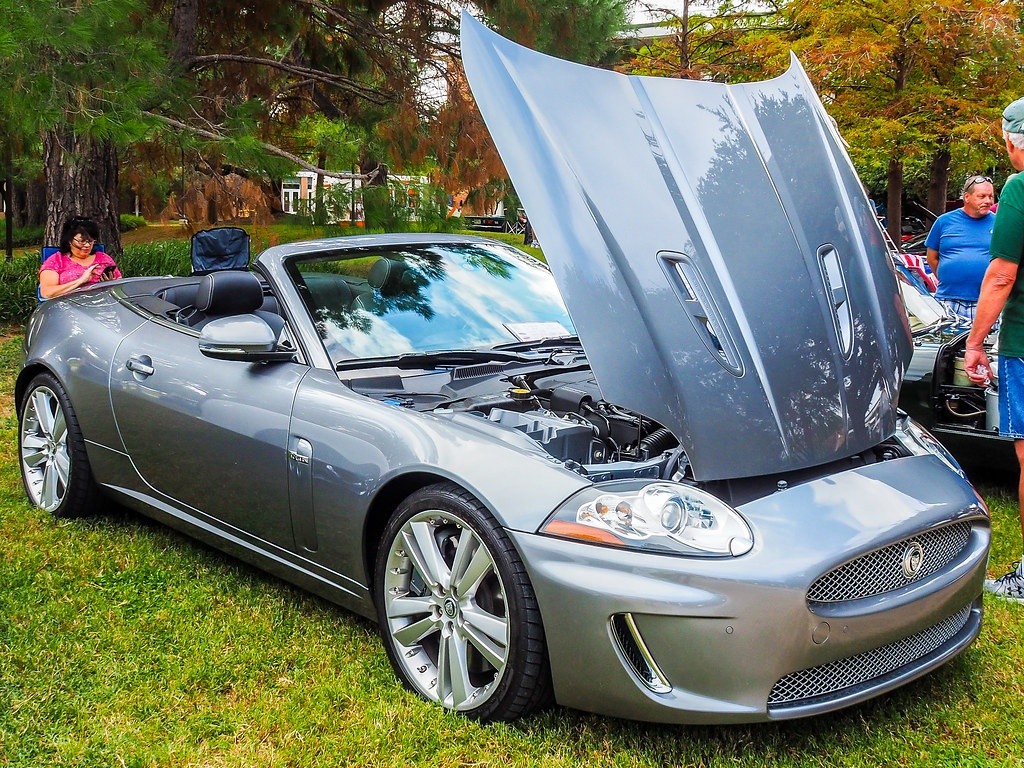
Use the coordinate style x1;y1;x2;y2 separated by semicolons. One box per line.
100;265;116;281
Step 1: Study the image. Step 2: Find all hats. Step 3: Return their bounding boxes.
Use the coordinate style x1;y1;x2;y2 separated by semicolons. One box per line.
1002;97;1024;134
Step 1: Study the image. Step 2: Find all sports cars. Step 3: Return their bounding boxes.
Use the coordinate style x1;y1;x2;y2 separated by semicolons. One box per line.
16;6;990;725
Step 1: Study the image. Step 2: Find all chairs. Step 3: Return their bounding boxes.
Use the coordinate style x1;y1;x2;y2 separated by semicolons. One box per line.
191;270;284;339
350;258;408;313
37;243;104;303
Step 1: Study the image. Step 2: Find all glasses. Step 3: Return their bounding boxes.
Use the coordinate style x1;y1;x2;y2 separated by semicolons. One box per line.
965;176;994;191
73;237;97;245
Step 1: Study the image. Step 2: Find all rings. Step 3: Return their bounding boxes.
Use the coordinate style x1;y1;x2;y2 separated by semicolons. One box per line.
968;377;971;381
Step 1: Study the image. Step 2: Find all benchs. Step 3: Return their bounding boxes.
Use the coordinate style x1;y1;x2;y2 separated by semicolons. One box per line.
162;276;352;317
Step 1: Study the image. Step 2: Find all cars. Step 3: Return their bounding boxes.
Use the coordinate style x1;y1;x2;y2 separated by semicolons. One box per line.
884;230;1016;451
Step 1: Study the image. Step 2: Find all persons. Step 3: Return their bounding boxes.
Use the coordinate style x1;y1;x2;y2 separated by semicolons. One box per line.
38;216;122;299
963;96;1024;605
834;204;914;371
924;173;1018;329
862;184;877;218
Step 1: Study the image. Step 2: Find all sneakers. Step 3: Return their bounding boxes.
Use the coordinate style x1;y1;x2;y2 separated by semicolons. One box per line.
985;561;1024;601
